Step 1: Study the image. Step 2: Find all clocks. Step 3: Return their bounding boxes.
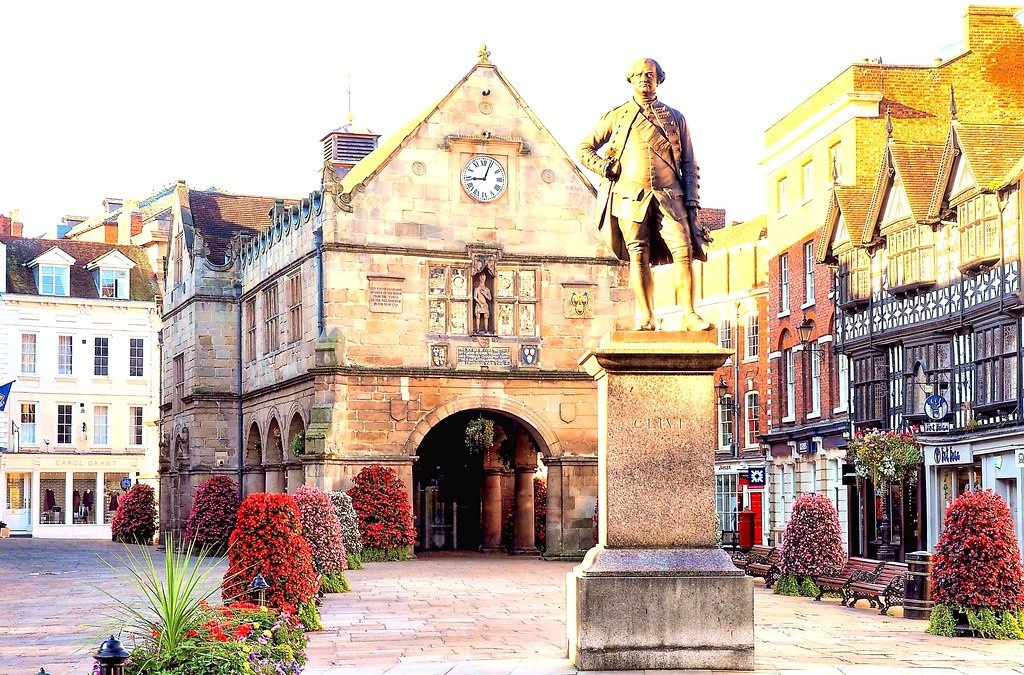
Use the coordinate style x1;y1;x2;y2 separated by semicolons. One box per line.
460;155;508;203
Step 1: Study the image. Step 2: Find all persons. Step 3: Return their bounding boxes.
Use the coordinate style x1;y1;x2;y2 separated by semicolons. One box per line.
577;57;716;331
44;488;120;514
474;274;491;333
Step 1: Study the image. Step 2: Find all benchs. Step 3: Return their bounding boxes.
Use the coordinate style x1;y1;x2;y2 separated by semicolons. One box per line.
730;545;784;588
816;557;908;616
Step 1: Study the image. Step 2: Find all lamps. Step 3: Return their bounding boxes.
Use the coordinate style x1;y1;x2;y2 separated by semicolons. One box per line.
714;378;734;408
796;314;825;361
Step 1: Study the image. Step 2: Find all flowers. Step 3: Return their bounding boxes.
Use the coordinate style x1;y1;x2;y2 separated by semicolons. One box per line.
846;425;925;484
